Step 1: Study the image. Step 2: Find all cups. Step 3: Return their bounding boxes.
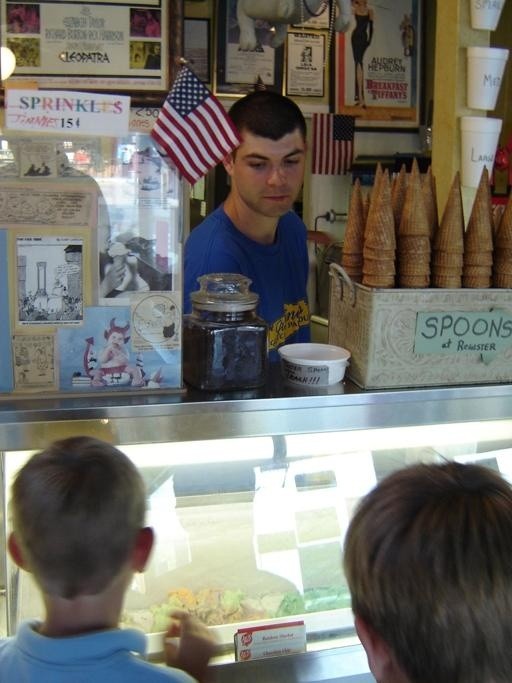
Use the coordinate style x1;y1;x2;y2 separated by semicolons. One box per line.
458;116;504;191
470;1;506;32
465;45;510;112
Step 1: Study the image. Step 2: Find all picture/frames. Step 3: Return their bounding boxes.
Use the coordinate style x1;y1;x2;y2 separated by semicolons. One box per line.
210;0;287;101
330;0;426;133
0;0;184;108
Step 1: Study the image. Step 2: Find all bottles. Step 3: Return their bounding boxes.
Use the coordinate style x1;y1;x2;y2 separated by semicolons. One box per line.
182;273;271;390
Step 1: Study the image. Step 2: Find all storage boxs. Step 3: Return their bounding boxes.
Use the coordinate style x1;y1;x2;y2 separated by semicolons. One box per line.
327;262;512;391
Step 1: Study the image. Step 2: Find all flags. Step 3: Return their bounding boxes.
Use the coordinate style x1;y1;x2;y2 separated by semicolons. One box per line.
311;112;355;176
150;61;244;187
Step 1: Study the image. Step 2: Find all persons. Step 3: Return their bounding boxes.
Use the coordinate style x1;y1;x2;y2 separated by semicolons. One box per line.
100;262;127;299
342;460;511;683
0;435;218;683
144;43;161;70
183;89;311;365
144;10;160;37
351;0;374;107
91;318;146;388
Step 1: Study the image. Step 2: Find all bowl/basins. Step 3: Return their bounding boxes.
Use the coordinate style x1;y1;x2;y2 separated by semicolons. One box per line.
276;341;353;387
